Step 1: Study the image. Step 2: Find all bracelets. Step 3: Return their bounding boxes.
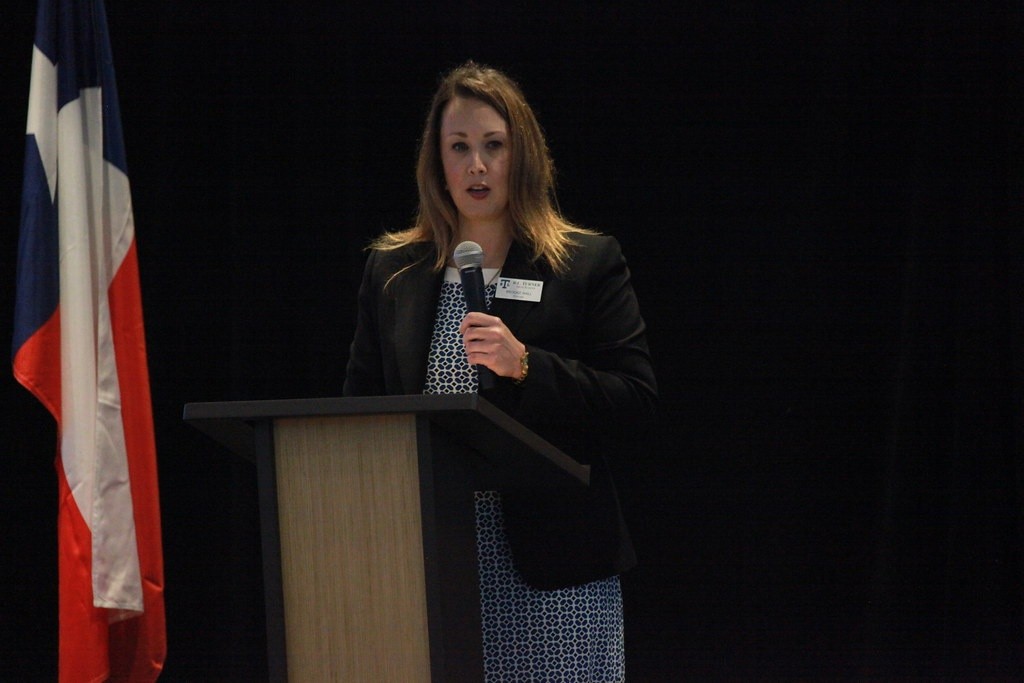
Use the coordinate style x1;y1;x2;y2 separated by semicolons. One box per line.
512;352;530;386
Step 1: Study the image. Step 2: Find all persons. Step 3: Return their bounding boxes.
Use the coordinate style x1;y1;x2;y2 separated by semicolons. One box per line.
343;66;659;683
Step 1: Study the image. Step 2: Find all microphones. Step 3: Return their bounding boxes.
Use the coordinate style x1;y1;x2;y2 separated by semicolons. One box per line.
453;240;488;369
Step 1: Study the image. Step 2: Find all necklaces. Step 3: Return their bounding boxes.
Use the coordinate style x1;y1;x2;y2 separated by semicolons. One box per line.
485;266;502;289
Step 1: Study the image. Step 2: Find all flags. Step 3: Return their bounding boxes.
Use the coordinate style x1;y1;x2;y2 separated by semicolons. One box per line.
10;0;168;683
